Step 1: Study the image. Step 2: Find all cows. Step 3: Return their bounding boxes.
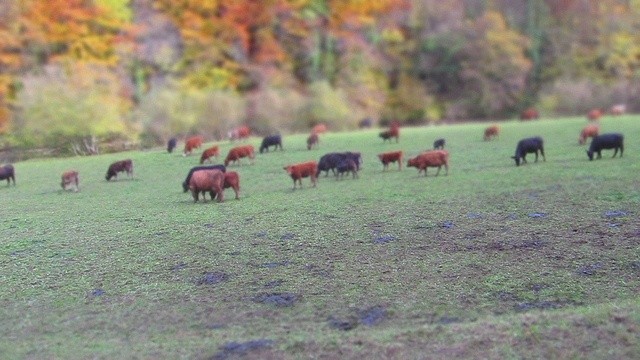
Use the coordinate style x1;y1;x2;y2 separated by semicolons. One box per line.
318;152;346;177
521;109;540;121
59;171;79;192
0;165;16;187
483;126;499;141
105;159;134;182
224;171;240;201
200;146;218;164
283;161;318;190
377;151;403;171
182;165;225;192
579;123;600;144
407;150;449;177
259;134;284;154
224;145;255;167
378;121;401;144
433;138;445;150
188;168;224;204
335;155;359;181
344;151;362;176
168;137;177;154
185;136;203;156
587;132;624;161
306;133;320;151
510;136;546;166
